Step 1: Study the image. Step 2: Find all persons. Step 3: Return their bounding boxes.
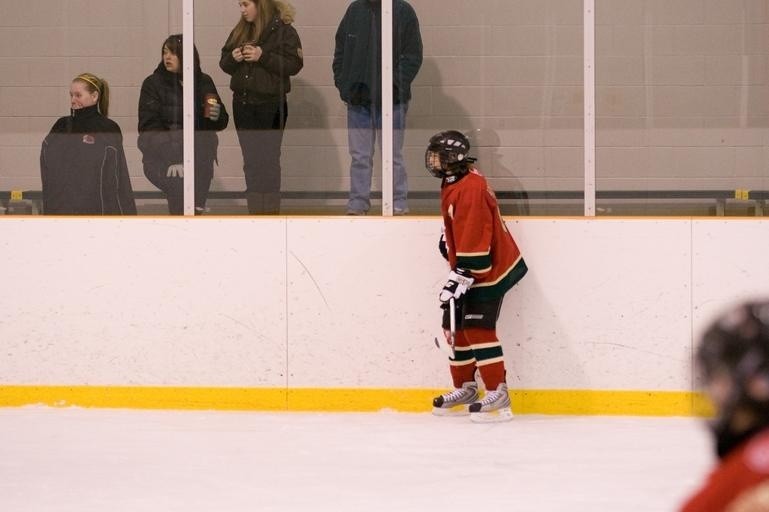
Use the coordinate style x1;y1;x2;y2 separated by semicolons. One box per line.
674;297;769;512
41;71;139;218
424;129;529;412
221;0;305;215
333;0;426;214
140;35;231;216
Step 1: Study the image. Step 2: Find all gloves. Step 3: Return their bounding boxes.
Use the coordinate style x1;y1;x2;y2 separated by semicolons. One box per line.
438;271;474;304
243;46;262;61
167;164;183;178
202;103;221;121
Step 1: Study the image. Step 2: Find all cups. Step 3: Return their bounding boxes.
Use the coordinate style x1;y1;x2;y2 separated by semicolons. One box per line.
205;93;218;117
240;43;258;62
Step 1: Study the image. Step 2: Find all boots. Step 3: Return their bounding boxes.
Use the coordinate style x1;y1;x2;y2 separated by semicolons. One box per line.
469;382;512;412
433;382;479;407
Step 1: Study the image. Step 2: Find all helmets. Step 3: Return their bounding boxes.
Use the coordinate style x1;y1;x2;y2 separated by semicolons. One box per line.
429;131;470;163
695;301;769;455
